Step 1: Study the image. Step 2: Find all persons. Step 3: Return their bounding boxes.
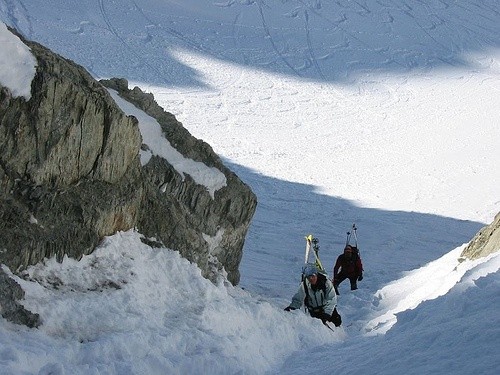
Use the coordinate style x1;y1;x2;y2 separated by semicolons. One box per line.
332;245;363;295
283;263;341;328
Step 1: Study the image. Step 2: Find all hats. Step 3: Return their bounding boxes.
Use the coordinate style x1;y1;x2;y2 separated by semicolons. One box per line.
306;262;318;278
345;246;352;253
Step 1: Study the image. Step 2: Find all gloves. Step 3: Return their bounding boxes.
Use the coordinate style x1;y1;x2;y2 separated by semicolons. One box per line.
320;313;330;324
358;275;363;281
283;306;296;312
